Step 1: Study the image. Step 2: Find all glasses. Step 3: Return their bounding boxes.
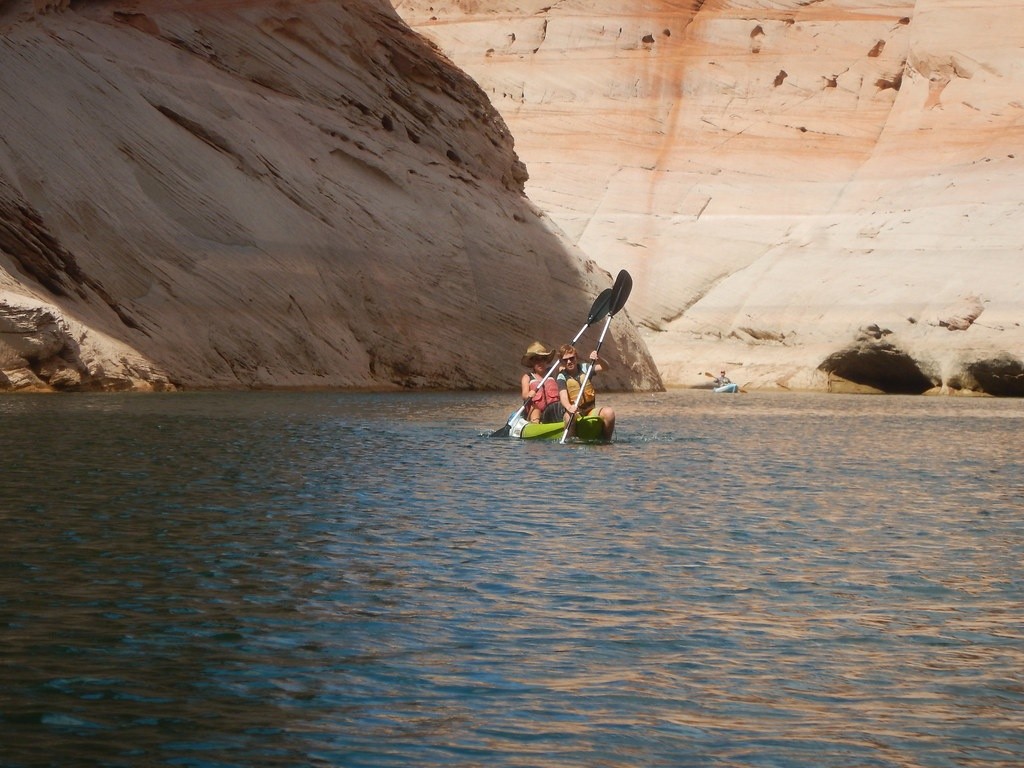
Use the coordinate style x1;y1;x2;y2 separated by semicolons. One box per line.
534;357;548;363
560;356;575;364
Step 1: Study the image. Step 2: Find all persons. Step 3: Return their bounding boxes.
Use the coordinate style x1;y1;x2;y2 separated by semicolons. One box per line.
521;342;565;423
713;371;731;387
556;344;616;444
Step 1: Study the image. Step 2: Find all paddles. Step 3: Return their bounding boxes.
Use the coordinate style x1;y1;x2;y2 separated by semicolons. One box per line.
492;288;612;437
560;268;634;444
705;372;747;393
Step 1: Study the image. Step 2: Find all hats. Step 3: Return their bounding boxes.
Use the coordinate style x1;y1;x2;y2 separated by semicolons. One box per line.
720;370;725;373
521;341;556;367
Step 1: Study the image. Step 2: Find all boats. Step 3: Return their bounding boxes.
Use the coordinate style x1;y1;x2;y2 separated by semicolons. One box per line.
713;383;738;393
505;411;605;439
